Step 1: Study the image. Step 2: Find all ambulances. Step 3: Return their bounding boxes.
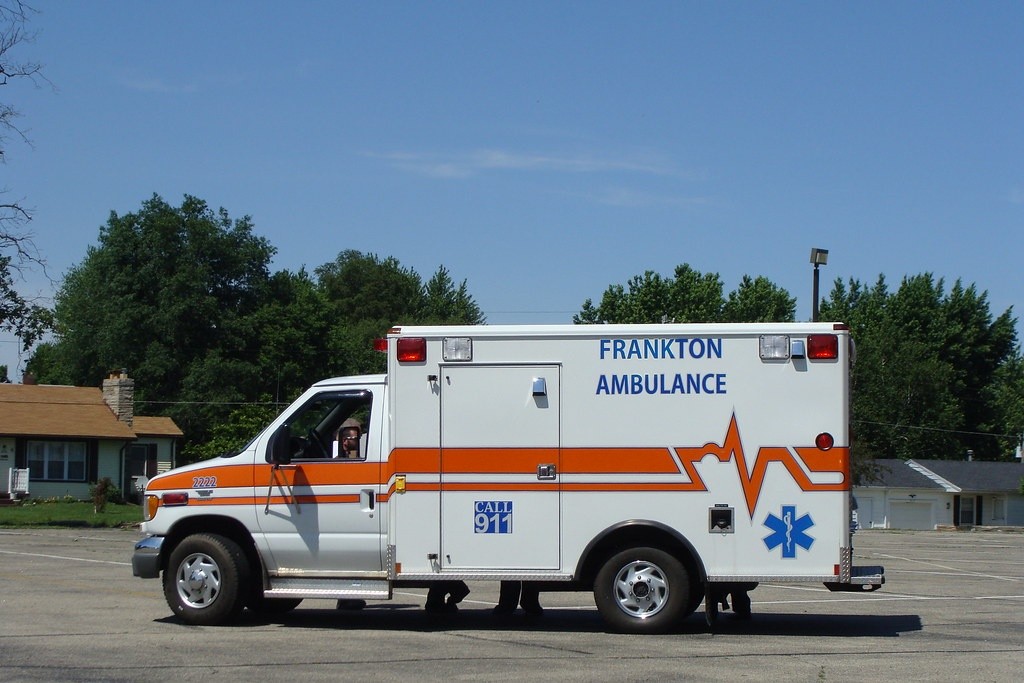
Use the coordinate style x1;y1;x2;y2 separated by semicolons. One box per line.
131;321;886;626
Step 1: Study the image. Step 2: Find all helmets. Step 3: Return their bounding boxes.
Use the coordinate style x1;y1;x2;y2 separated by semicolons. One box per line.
338;418;361;440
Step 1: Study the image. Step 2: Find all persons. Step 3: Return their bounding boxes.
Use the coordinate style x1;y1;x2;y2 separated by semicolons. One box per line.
343;428;358;459
331;579;758;617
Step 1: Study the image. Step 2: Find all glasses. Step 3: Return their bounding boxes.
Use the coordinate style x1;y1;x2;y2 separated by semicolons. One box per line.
343;434;360;440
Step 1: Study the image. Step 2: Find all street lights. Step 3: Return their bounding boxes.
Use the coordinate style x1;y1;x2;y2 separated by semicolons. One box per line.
810;246;830;321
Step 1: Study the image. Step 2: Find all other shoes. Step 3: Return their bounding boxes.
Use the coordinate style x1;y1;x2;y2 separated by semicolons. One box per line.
520;596;544;616
734;611;748;628
706;608;718;627
425;594;450;625
448;582;470;604
493;599;518;616
